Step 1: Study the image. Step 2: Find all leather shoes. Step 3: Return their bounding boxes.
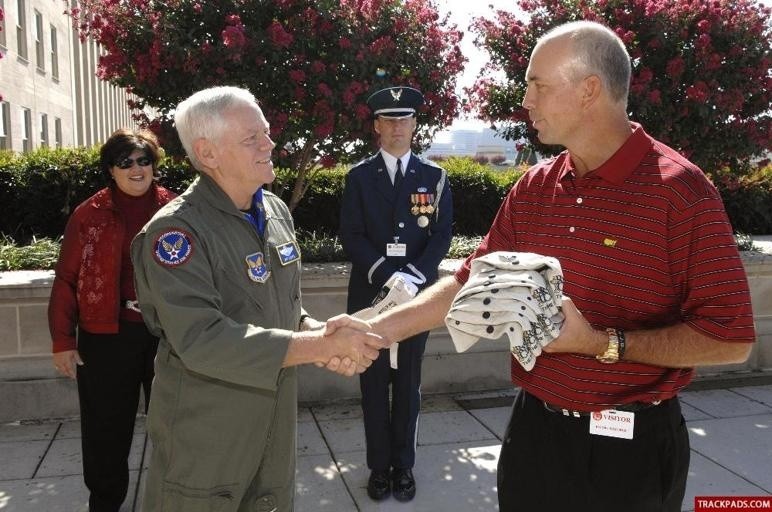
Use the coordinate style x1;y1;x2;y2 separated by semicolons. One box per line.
368;466;415;501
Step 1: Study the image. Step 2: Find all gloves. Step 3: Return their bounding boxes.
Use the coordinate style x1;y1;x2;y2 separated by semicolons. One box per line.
386;271;422;297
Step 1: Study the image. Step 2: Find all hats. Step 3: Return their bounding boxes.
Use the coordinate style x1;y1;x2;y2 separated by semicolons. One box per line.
366;87;424;120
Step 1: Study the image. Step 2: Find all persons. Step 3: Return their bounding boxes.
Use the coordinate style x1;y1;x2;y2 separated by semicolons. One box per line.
316;21;756;512
129;86;393;512
48;128;180;512
339;85;453;502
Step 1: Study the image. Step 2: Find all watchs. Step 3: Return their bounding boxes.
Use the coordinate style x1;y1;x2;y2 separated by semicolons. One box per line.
596;327;620;363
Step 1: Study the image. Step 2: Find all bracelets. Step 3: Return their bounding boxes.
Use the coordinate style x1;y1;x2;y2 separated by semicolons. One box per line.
299;315;311;331
618;328;626;360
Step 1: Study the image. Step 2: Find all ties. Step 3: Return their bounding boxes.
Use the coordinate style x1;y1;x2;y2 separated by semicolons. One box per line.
394;159;406;201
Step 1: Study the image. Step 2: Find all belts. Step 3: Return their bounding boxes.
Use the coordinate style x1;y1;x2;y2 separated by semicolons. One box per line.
522;389;662;419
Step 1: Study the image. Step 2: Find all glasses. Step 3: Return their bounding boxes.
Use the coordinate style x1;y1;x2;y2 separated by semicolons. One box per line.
115;157;152;170
